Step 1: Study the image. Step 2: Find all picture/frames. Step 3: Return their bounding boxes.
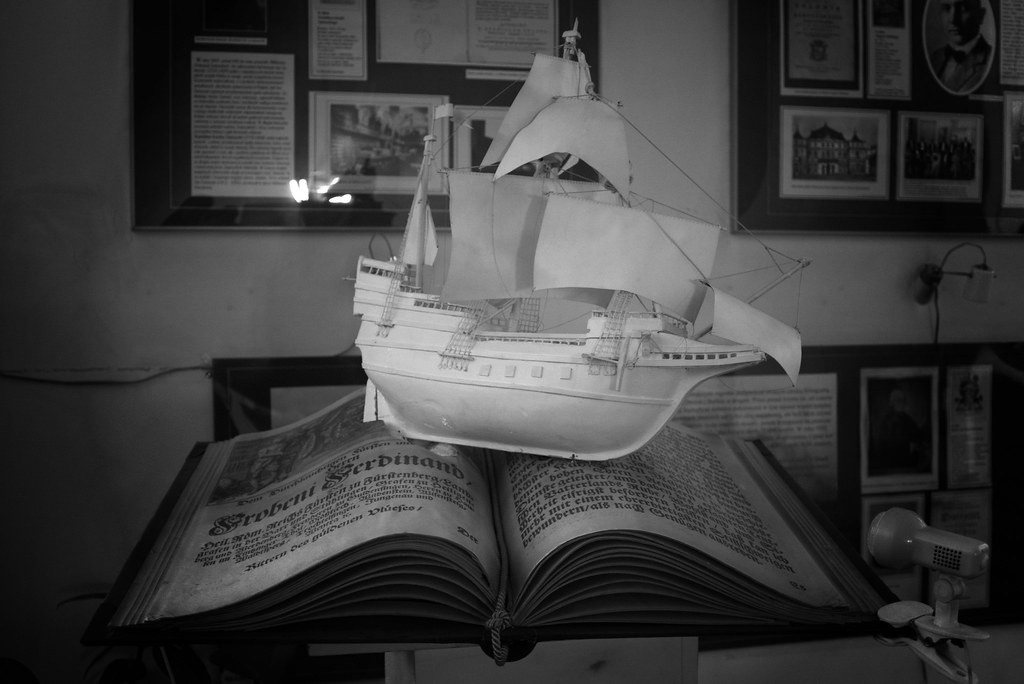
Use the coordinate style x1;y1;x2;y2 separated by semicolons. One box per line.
205;341;1024;652
725;1;1024;243
126;2;602;239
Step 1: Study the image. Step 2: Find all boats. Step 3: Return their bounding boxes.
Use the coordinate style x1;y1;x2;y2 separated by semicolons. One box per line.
335;13;820;465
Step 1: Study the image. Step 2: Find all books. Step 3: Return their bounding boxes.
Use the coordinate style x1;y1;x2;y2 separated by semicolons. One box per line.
77;382;919;665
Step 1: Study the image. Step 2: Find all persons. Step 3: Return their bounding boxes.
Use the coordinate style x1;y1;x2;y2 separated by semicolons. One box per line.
869;382;930;473
930;0;994;93
